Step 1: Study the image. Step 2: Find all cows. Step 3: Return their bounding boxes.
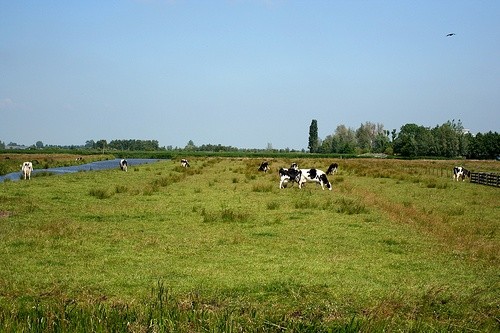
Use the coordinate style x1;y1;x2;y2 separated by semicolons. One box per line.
258;160;270;173
453;166;470;182
22;162;33;180
279;163;332;191
120;159;128;173
180;159;190;168
326;164;338;175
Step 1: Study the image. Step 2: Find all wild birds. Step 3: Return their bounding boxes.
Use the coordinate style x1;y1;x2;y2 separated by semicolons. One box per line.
446;33;455;37
